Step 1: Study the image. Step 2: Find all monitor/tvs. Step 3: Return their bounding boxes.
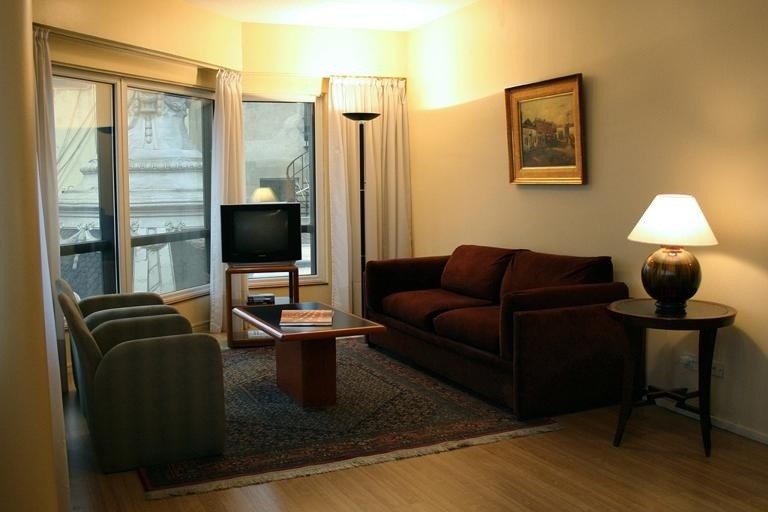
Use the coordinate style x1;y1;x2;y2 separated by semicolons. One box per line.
222;204;303;270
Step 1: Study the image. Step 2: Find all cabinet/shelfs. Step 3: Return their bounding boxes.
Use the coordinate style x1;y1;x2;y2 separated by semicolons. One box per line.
225;264;299;349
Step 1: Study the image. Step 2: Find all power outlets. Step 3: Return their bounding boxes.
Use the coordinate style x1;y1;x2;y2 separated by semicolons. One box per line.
679;352;699;371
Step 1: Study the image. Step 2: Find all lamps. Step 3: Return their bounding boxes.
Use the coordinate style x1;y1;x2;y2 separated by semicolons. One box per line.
342;111;381;344
627;194;719;315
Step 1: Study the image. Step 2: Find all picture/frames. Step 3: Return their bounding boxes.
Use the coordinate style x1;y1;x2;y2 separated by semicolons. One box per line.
504;72;588;187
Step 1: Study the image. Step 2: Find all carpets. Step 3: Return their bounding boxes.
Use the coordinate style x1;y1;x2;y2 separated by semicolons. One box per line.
134;336;568;502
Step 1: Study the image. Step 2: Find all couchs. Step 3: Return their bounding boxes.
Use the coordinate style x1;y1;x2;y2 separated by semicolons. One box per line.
362;245;648;422
57;293;226;474
53;277;181;396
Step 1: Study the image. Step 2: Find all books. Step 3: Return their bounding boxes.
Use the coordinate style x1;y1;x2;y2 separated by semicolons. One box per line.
278;307;333;325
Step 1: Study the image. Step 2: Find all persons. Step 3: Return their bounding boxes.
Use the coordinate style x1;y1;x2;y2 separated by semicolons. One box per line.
568;134;574;147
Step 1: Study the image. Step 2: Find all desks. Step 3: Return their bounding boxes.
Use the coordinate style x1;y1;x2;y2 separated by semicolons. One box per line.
604;296;737;458
